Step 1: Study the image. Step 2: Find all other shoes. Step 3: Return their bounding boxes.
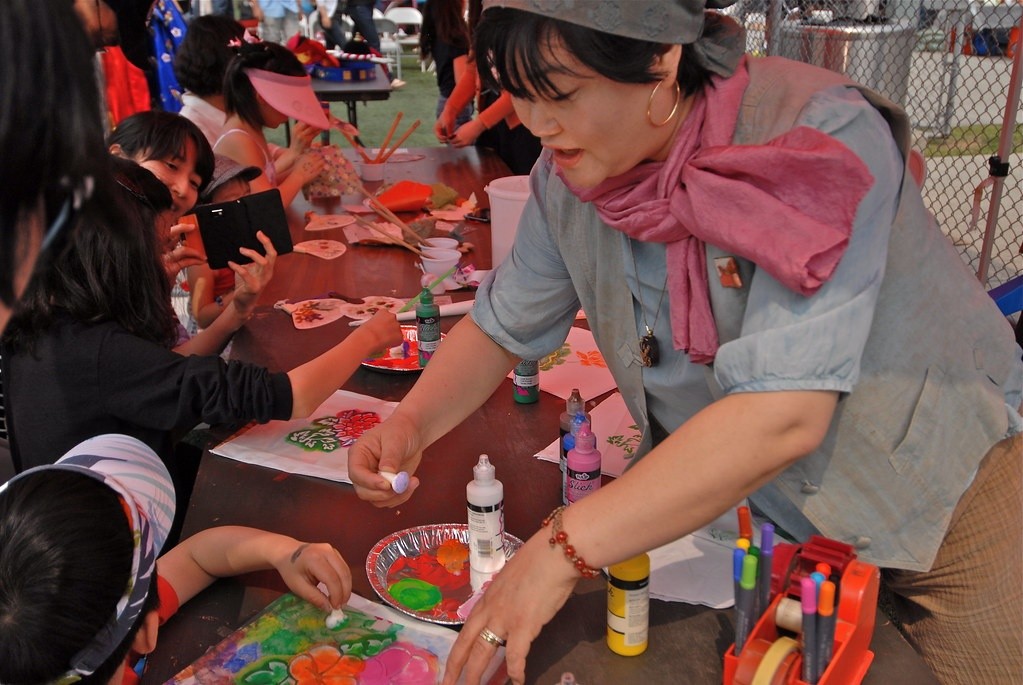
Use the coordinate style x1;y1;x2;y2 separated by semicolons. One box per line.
389;78;405;88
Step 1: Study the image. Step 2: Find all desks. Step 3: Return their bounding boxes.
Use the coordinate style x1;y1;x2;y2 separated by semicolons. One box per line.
140;144;936;685
284;63;392;148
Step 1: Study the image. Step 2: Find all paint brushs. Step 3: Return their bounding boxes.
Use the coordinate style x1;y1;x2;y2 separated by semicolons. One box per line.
343;174;435;247
379;120;422;164
374;111;402;164
361;217;434;259
340;131;371;163
399;261;465;313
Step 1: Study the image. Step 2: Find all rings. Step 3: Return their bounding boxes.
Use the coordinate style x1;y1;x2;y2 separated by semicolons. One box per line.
480;627;505;647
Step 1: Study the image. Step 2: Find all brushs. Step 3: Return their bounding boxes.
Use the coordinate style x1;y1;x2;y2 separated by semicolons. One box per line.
325;112;359;135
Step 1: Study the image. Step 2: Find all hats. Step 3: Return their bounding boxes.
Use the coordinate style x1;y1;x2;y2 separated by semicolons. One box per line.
0;434;179;685
201;150;261;200
240;66;330;131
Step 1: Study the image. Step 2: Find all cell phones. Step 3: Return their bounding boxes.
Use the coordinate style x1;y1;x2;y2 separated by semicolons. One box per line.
196;200;258;268
464;207;490;222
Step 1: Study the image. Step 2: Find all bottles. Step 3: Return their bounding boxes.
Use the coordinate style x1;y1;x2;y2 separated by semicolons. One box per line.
558;388;602;506
415;289;440;366
606;554;651;656
513;358;539;403
467;454;506;573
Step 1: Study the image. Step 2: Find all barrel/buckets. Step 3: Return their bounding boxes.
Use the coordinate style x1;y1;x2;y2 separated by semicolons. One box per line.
485;175;532;269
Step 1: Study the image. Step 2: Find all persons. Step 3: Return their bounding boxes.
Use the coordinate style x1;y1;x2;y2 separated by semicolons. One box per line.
378;0;544;179
0;434;353;685
349;0;1022;684
0;1;404;552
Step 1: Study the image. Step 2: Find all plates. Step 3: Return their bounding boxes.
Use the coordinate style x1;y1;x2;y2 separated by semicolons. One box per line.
360;324;448;371
366;522;525;624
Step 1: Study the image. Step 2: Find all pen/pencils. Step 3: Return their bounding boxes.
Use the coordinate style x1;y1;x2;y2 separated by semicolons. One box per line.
733;549;746;614
749;546;761;560
810;573;825;610
737;506;753;545
829;574;841;635
759;523;775;617
816;580;836;681
816;563;832;580
734;554;758;657
736;539;751;554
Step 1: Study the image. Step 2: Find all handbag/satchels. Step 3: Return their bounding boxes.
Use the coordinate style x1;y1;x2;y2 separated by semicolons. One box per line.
89;44;153;147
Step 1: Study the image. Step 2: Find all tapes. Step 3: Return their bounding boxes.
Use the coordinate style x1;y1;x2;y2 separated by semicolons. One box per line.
749;636;804;685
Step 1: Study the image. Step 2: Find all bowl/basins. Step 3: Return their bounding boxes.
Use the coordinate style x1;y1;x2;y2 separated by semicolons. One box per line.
358;160;385;182
419;248;462;275
417;237;459;251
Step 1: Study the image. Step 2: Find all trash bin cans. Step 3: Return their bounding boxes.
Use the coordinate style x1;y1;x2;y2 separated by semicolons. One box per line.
780;15;916;109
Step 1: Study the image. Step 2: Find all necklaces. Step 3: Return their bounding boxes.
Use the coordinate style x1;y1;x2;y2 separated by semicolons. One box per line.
621;235;667;369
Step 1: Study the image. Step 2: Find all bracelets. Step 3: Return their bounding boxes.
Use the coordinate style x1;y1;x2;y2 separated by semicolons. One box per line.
540;505;602;582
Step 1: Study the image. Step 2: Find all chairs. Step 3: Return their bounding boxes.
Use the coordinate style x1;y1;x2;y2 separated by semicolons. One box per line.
371;7;426;82
